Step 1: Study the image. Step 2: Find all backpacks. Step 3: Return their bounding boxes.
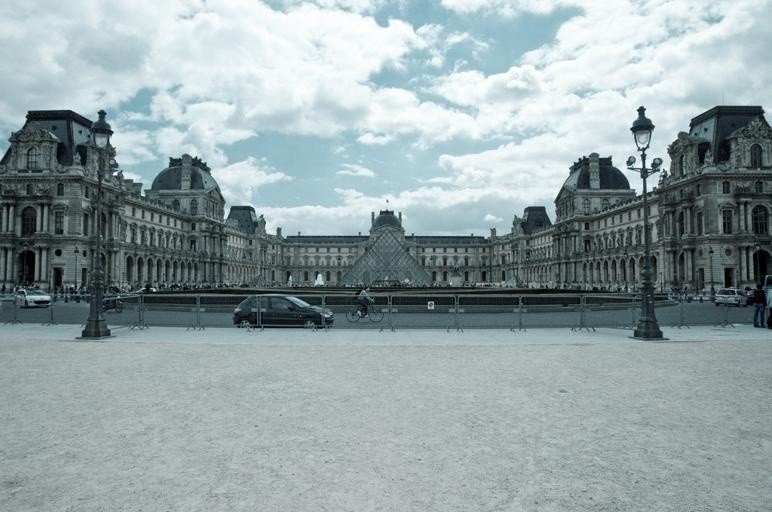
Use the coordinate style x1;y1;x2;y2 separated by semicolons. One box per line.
354;290;363;300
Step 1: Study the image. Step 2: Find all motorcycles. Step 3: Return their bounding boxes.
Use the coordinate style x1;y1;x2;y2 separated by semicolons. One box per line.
102;293;123;314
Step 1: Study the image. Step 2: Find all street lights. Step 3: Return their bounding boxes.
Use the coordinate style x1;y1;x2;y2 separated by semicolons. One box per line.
754;238;762;289
80;109;116;340
525;256;530;288
707;247;715;302
627;106;669;341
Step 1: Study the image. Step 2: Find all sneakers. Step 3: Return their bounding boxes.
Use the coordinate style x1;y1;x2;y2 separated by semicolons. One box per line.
358;310;361;316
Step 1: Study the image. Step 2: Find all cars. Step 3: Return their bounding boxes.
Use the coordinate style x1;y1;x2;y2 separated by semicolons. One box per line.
232;294;335;330
714;288;747;307
130;287;156;293
13;287;53;310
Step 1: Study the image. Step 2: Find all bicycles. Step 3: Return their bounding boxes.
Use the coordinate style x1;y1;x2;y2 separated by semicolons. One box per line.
345;297;385;323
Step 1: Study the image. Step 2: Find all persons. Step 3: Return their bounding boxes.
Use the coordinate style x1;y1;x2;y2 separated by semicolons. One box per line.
357;286;375;316
752;283;766;328
766;289;772;329
1;282;88;303
